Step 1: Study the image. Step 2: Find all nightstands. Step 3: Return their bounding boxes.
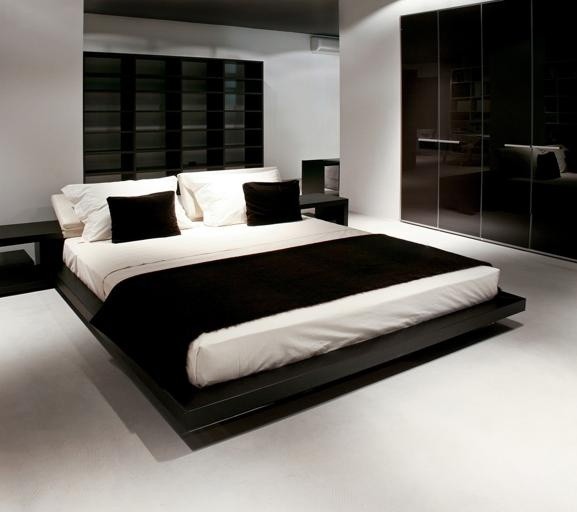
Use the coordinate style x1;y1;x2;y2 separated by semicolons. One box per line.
0;220;65;301
298;192;350;227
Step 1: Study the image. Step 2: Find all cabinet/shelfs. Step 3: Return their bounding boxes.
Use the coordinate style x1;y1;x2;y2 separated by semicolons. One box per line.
446;68;489;131
539;58;577;126
81;48;266;182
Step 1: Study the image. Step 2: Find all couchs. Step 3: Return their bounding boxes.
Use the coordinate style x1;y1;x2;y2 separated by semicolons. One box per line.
495;143;577;220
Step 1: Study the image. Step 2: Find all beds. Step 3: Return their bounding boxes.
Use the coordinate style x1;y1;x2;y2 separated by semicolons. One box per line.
49;166;526;437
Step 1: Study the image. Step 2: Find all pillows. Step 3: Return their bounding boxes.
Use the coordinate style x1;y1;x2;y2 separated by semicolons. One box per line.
57;167;303;247
504;146;566;180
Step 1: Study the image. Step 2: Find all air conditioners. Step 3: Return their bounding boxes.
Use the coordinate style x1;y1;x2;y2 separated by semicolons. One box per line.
308;33;339;55
